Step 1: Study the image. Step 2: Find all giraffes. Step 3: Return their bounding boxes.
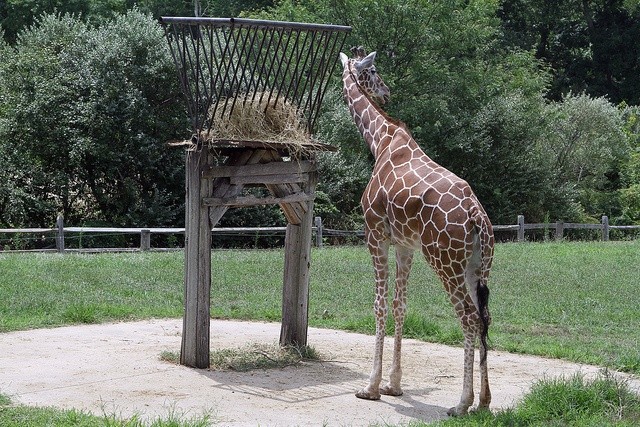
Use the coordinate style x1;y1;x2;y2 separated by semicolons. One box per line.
339;45;495;421
